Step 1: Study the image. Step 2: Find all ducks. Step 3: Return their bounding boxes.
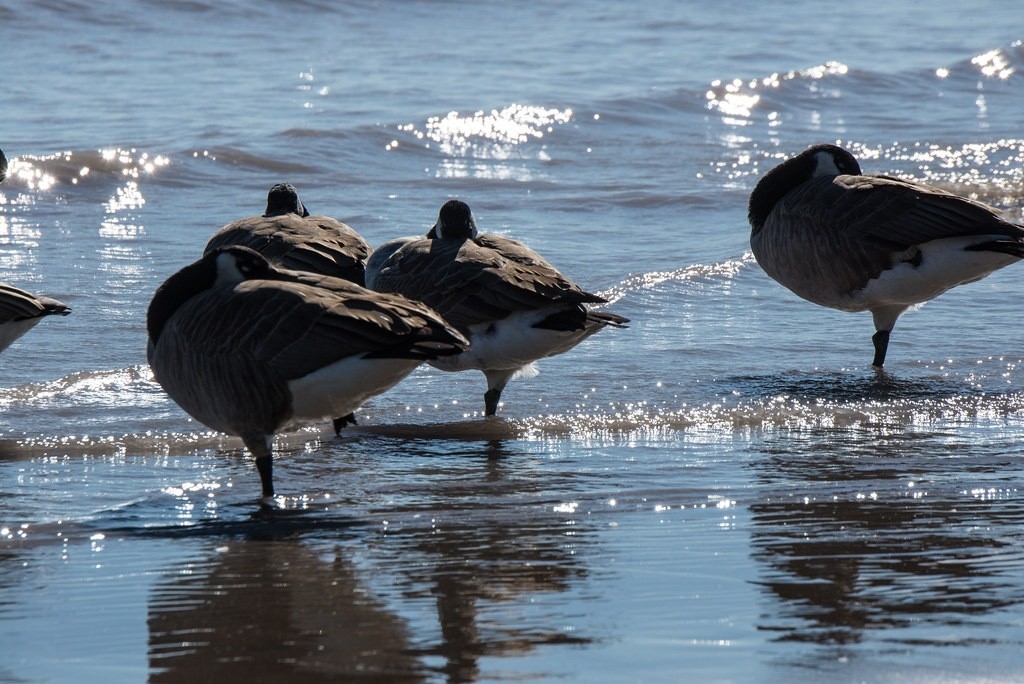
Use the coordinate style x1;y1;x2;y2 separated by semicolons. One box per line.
366;201;629;416
746;144;1024;366
0;148;72;354
147;248;472;496
203;185;373;436
